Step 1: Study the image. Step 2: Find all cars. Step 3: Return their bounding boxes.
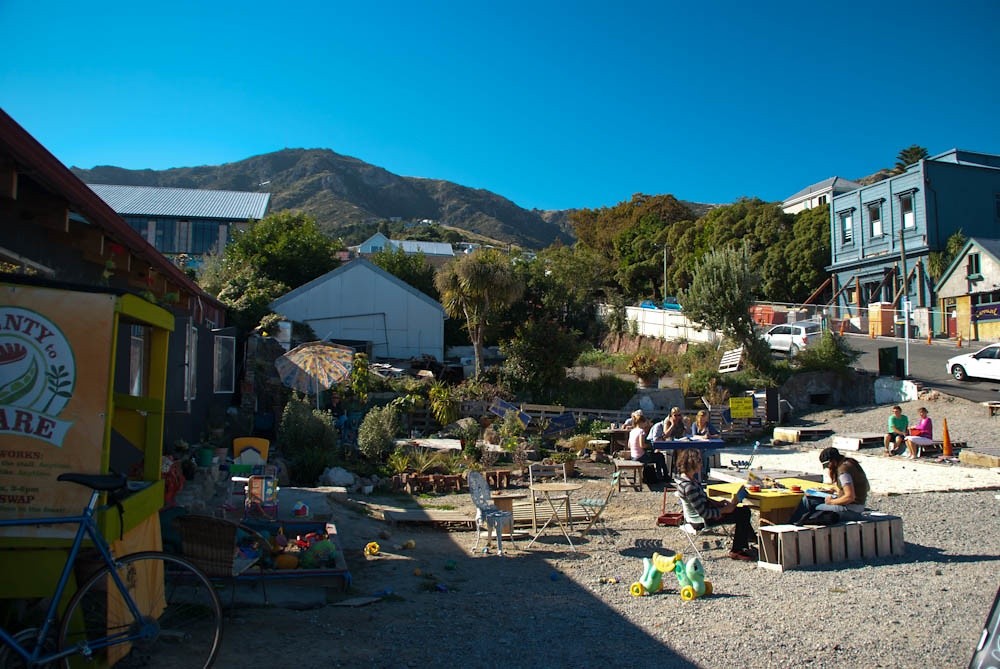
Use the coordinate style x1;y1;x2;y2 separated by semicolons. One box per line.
641;300;662;309
946;342;1000;382
758;321;822;356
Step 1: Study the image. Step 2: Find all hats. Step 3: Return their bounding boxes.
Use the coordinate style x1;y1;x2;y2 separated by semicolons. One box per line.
819;447;838;469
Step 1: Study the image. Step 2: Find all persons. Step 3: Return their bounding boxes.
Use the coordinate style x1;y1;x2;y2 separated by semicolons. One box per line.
789;448;870;525
676;448;761;561
621;407;721;483
884;406;932;459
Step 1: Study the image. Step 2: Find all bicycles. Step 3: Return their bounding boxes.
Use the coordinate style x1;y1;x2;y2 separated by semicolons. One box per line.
0;472;221;669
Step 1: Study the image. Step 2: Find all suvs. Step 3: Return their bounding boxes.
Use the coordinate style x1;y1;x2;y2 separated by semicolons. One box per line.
662;297;682;310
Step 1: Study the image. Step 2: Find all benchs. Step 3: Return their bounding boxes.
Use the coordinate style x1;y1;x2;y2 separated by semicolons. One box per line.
757;515;904;571
614;457;643;492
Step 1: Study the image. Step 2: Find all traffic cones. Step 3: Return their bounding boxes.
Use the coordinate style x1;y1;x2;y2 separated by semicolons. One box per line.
838;321;845;336
869;323;877;339
888;320;896;336
939;418;959;458
955;331;964;348
925;328;933;345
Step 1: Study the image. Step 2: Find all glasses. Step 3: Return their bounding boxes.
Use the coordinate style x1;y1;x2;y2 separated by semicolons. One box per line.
672;414;681;416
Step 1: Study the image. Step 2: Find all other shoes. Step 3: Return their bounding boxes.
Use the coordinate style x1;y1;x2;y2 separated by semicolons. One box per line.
728;551;753;560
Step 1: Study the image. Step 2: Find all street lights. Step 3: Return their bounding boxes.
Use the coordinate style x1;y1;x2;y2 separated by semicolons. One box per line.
880;232;927;376
664;245;671;299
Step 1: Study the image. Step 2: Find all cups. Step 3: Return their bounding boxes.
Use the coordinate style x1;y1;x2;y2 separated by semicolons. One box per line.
792;485;800;491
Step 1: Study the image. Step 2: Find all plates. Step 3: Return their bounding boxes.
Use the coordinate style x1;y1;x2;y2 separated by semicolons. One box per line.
791;491;803;493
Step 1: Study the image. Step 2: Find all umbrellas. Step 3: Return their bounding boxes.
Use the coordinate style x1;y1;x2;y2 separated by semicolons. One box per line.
274;340;357;411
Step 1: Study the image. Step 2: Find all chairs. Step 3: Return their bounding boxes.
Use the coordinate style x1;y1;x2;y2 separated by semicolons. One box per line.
172;512;267;623
674;490;709;562
467;472;518;558
580;488;616;545
528;464;573;532
731;445;758;472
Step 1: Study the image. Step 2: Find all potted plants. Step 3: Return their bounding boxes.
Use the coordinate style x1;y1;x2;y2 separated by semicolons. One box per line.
552;453;578;473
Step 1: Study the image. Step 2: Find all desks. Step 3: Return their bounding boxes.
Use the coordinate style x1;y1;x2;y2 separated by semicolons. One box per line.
650;438;723;481
706;477;835;527
527;483;583;553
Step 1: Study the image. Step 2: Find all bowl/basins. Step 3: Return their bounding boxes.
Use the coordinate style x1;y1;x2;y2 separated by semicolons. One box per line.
908;428;921;436
